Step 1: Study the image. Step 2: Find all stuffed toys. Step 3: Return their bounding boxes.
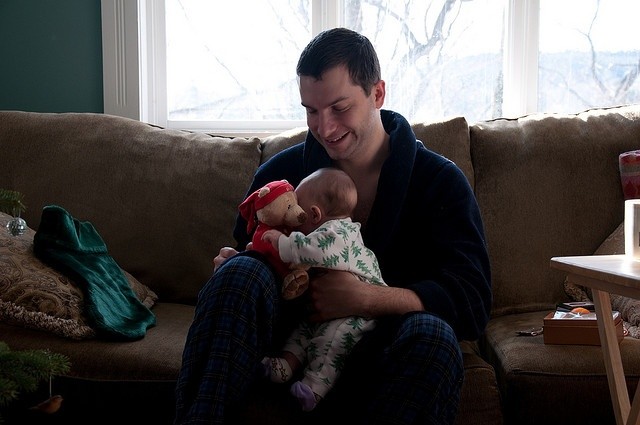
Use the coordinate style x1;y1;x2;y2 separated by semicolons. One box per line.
237;177;313;302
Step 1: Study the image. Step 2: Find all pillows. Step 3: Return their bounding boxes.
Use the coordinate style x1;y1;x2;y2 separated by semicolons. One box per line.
562;219;639;339
0;210;159;344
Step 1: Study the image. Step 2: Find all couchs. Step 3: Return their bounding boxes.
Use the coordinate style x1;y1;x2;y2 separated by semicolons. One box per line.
1;104;639;425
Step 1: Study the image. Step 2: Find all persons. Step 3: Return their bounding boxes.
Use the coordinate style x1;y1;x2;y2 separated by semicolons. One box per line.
166;26;495;424
257;163;391;412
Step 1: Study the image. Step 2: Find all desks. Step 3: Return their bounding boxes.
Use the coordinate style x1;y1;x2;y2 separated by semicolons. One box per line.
546;254;640;425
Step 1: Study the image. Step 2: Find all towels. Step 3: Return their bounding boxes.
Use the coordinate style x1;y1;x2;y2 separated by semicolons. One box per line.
32;204;156;339
